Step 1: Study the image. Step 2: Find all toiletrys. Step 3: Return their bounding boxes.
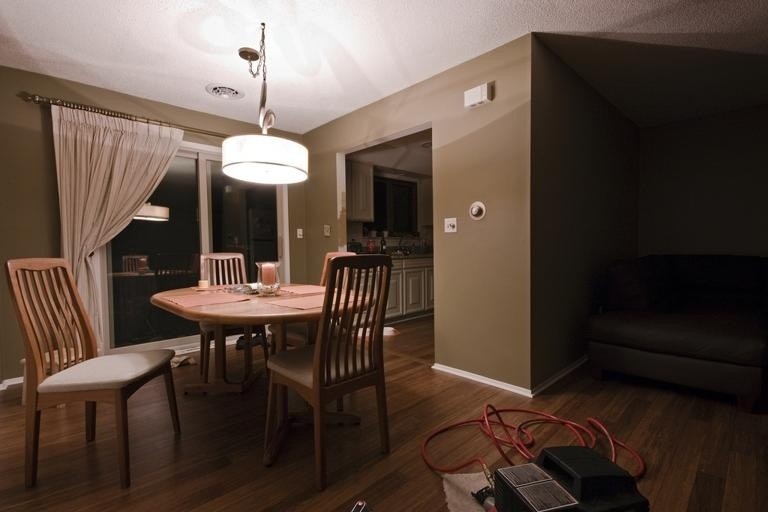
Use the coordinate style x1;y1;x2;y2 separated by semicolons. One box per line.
380;230;390;253
367;230;379;253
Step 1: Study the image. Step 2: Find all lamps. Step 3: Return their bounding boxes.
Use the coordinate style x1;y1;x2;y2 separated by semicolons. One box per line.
221;18;311;186
133;202;171;223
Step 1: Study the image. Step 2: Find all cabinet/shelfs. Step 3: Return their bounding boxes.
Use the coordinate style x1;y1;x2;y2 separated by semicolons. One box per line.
355;260;433;322
345;160;374;223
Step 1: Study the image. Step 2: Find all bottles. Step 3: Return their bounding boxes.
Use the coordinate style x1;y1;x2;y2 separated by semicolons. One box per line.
380;232;387;254
256;261;281;296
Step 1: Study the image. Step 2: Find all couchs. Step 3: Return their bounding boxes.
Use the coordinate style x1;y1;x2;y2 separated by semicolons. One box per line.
585;250;766;412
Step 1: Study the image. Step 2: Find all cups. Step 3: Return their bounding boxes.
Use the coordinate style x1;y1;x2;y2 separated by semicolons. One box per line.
198;280;209;289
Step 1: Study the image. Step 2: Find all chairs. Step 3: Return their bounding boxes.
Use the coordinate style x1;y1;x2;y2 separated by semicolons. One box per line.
262;252;400;493
6;255;181;491
122;258;180;342
199;249;268;383
